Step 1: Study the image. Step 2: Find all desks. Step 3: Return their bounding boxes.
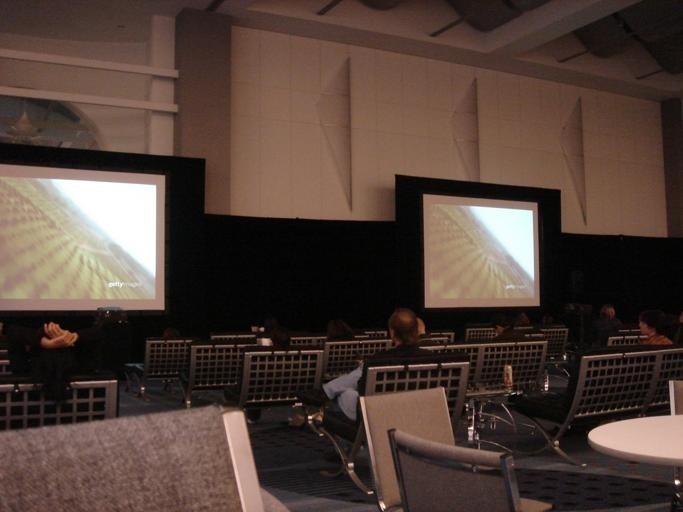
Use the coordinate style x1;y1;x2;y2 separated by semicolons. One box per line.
587;412;682;510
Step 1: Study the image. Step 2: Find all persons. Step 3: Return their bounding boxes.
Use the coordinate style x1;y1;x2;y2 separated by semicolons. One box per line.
600;303;625;337
490;316;530;361
246;325;291;425
638;309;676;345
416;317;435;347
298;305;422;461
320;317;358;375
4;319;104;404
262;313;281;336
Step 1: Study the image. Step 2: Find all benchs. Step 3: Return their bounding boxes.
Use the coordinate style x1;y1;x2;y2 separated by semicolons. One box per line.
484;347;681;466
291;361;472;495
1;380;121;428
2;325;681;433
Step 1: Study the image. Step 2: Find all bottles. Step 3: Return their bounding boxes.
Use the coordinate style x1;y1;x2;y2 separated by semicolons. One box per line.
503;361;514;389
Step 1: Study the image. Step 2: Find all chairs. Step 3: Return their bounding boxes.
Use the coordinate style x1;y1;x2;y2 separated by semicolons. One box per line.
2;405;291;511
391;428;555;511
359;385;456;508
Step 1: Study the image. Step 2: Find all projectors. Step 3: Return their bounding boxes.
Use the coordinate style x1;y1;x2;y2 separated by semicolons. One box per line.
560;302;593;315
92;306;129;329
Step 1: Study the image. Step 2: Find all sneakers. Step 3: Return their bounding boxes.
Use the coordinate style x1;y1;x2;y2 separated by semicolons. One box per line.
295;389;324;407
